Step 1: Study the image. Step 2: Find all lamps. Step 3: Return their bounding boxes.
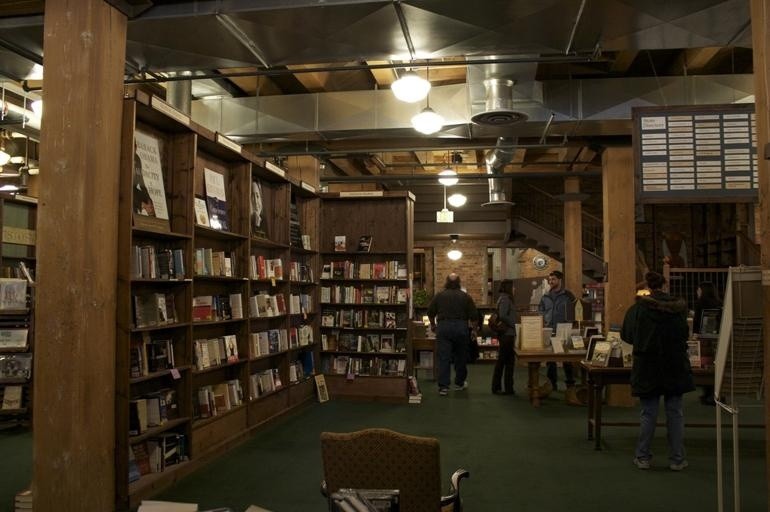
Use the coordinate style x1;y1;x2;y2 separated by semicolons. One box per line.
0;96;42;199
445;241;466;262
390;59;467;208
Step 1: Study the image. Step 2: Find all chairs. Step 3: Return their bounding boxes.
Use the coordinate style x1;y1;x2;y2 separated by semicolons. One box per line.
320;429;470;512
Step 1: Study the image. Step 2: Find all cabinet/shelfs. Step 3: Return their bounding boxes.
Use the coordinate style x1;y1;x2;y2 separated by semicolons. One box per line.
290;245;319;385
193;222;249;429
320;250;410;380
249;234;289;399
129;227;193;495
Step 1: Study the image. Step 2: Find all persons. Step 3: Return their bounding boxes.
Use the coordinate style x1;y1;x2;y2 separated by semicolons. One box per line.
428;273;478;396
620;271;691;471
537;270;579;390
492;278;519;397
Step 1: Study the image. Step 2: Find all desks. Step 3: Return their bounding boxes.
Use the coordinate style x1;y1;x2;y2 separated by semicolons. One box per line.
581;359;714;452
513;346;587;406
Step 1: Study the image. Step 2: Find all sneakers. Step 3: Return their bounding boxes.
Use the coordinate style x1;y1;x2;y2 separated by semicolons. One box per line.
440;389;449;396
671;461;689;471
632;458;650;469
455;381;468;390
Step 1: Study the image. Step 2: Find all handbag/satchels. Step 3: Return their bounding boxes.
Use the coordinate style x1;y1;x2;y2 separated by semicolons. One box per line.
487;313;507;334
463;339;479;364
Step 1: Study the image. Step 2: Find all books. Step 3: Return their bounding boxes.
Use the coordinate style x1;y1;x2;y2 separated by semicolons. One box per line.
2;260;34;410
315;374;328;403
129;236;315;484
15;489;33;511
551;323;633;368
476;313;499;360
319;235;408;377
409;376;422;404
